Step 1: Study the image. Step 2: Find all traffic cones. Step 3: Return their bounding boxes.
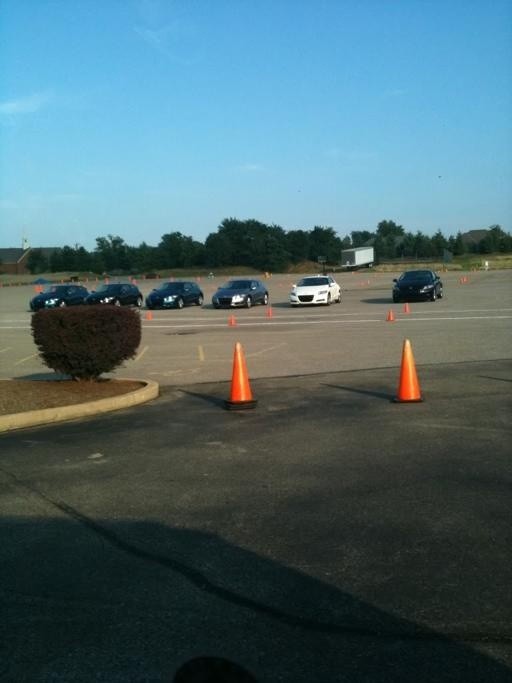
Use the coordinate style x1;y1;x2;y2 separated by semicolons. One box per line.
386;303;409;321
223;340;258;411
146;310;153;320
391;336;423;403
228;306;272;327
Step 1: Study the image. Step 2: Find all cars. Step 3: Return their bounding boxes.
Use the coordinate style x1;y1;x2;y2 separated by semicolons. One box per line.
30;284;90;311
288;275;343;308
83;283;143;309
392;269;442;303
211;279;270;309
145;281;204;310
